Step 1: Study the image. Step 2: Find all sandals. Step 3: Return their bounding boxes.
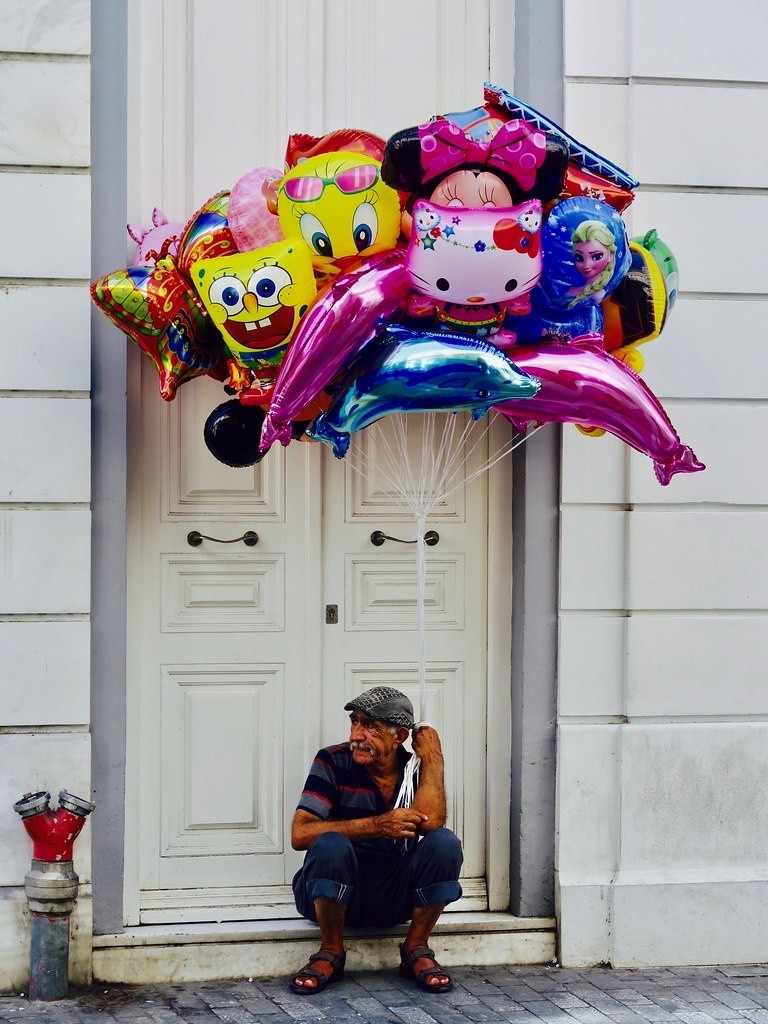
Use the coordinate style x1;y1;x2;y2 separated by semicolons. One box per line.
290;951;347;995
397;943;453;994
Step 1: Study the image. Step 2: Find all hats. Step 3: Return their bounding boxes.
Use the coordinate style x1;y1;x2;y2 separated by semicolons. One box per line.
343;686;414;729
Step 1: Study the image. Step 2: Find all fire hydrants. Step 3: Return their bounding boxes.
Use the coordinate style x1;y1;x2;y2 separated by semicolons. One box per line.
12;790;96;1004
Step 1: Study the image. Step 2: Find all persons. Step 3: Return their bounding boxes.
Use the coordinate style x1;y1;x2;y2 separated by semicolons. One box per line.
290;686;465;995
